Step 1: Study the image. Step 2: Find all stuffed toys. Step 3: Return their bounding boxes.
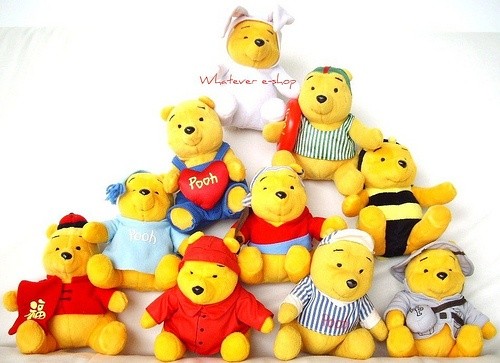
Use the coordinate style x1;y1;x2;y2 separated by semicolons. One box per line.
4;213;128;356
274;228;388;361
224;163;347;284
342;137;457;257
200;5;300;131
140;231;274;362
383;239;497;357
82;170;203;292
262;66;383;196
160;96;250;235
